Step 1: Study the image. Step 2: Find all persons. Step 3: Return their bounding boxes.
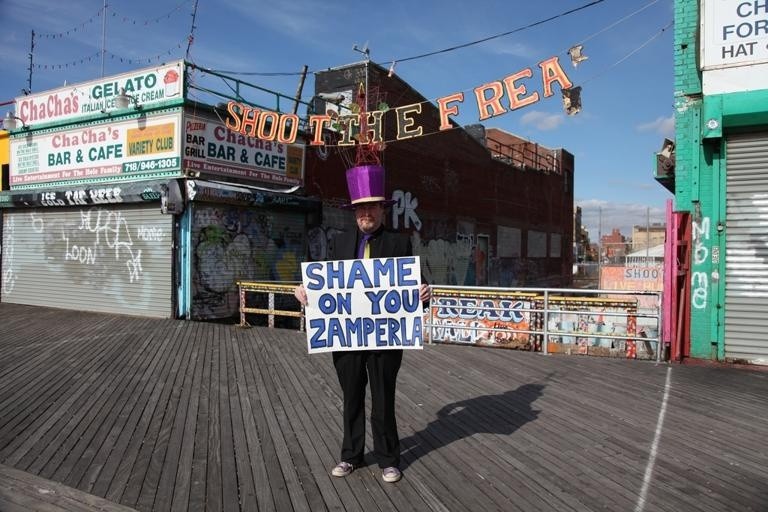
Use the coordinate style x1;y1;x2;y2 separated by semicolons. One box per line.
294;205;433;483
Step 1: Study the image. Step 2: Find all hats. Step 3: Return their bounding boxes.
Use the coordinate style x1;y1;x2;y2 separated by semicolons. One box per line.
339;167;398;210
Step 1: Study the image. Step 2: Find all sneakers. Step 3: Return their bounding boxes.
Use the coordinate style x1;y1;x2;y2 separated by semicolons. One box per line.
383;466;401;484
332;461;355;477
